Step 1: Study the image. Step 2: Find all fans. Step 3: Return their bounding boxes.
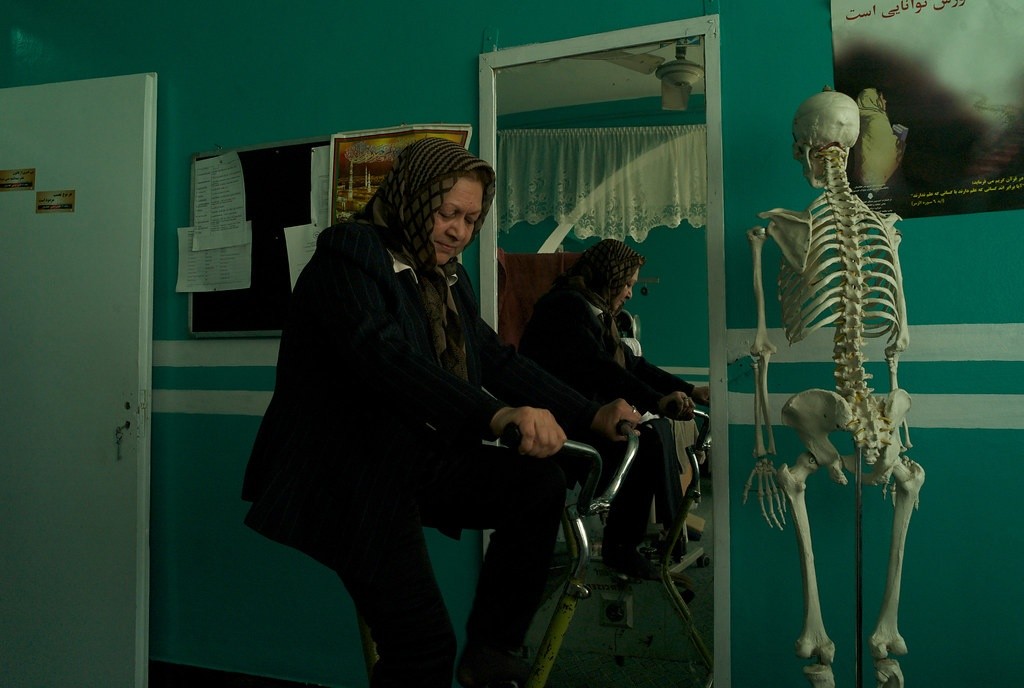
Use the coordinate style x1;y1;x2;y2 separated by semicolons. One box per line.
577;46;706;110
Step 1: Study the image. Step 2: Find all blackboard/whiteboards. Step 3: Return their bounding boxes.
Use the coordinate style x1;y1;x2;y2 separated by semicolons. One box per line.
187;134;334;339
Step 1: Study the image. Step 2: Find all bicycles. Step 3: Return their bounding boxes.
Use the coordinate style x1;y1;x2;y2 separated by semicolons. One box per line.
606;404;718;677
352;406;643;688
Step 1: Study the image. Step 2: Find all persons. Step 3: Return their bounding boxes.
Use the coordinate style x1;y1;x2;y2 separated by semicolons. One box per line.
242;136;641;688
518;238;711;586
743;91;926;688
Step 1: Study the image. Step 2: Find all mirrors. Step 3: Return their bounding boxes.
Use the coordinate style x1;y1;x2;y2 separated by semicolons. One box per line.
479;14;734;688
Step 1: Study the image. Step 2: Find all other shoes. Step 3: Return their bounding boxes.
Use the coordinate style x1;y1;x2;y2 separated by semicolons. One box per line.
455;641;531;688
601;547;660;579
650;538;686;563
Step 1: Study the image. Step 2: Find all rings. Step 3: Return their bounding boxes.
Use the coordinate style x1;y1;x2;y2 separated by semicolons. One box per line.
631;404;637;413
684;398;689;400
